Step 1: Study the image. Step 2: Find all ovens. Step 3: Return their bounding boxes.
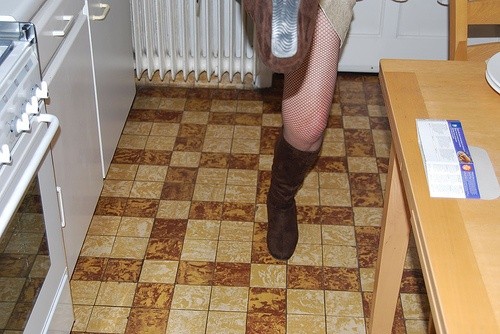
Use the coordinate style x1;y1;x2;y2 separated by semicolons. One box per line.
0;102;76;334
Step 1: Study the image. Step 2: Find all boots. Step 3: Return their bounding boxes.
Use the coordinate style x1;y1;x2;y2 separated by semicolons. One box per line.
266;135;323;261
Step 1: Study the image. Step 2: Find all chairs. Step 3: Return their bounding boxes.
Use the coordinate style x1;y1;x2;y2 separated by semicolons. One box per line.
449;0;500;60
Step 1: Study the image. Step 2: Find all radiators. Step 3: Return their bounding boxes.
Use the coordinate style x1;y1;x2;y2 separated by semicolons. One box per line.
129;0;260;83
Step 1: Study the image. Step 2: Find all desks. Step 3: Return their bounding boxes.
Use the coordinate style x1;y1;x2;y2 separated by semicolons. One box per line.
365;60;500;334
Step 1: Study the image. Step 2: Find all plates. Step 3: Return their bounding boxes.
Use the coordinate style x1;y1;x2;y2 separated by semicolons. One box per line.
485;52;500;95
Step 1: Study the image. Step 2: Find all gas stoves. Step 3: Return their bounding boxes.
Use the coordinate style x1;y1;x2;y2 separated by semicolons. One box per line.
0;21;49;172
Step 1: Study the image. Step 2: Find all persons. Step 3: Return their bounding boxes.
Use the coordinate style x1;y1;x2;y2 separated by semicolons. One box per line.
235;0;357;260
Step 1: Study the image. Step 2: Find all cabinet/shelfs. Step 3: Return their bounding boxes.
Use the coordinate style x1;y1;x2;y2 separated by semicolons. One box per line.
28;0;137;282
